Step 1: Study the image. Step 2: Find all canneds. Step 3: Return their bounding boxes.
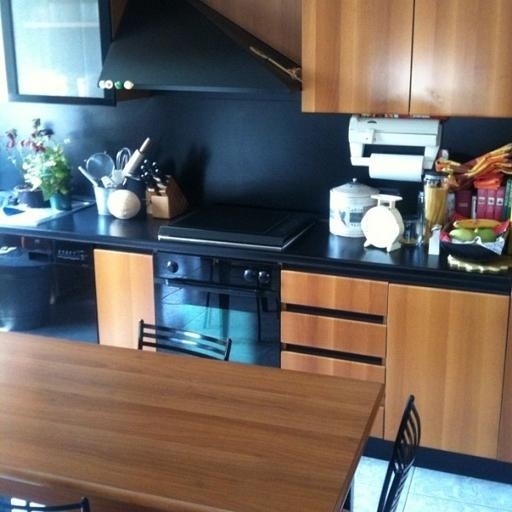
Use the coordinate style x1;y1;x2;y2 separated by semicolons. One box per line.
423;172;448;241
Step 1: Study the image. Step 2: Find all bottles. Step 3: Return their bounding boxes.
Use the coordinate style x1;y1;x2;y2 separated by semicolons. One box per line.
421;170;448;246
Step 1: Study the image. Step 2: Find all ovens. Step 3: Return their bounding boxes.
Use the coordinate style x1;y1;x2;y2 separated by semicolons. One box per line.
151;250;282;369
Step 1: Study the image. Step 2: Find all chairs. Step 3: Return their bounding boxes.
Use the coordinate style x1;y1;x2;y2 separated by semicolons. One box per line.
131;315;235;365
353;386;426;511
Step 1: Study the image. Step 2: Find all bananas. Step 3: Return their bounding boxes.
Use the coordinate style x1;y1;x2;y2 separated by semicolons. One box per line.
453;218;500;229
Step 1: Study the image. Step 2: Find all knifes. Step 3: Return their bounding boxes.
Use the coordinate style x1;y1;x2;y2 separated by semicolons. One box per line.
137;157;169;191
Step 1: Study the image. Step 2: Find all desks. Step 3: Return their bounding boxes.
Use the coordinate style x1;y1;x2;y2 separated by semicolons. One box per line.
2;318;386;511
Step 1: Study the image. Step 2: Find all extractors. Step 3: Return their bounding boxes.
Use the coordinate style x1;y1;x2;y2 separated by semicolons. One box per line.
97;0;303;104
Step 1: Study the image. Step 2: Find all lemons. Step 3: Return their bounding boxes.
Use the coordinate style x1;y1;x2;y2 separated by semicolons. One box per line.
448;227;496;241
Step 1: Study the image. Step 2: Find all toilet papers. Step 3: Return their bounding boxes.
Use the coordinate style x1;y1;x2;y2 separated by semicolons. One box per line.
368;153;425;183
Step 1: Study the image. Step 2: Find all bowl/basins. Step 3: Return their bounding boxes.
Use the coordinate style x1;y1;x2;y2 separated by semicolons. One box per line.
438;215;511;264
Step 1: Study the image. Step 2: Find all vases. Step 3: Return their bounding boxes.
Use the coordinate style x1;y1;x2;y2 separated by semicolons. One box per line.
48;190;78;213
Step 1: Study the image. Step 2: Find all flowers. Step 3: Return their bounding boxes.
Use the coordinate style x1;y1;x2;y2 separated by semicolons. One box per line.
2;116;75;207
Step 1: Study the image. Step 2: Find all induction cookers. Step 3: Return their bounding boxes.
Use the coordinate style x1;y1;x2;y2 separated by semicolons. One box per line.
156;201;317;251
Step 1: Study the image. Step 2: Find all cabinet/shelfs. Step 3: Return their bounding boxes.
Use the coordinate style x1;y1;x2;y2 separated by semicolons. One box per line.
384;284;509;469
277;266;388;445
298;0;512;121
88;249;155;350
